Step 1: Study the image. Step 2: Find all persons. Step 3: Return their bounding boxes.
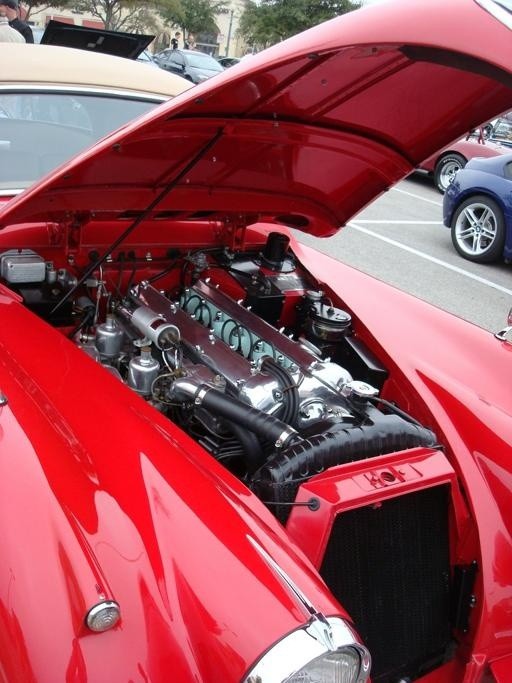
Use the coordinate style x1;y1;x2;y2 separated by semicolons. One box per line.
171;32;196;50
0;0;34;44
466;125;485;144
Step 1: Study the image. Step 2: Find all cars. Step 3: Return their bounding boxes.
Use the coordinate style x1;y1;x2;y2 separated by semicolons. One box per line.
135;50;160;68
217;57;241;68
418;136;512;195
28;26;46;44
442;152;512;265
0;0;512;683
151;47;225;85
